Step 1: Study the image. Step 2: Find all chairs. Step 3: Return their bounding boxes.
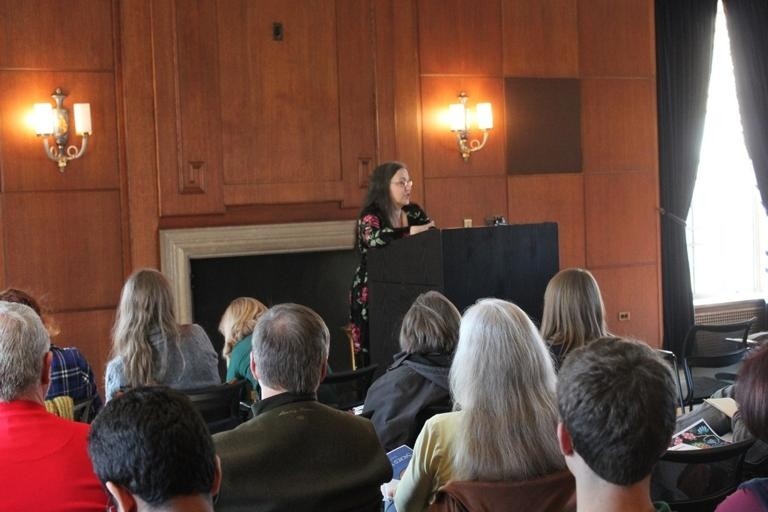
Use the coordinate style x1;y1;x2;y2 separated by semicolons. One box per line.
43;314;768;511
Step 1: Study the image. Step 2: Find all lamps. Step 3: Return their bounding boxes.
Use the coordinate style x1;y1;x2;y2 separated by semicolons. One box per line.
446;87;493;162
28;84;91;172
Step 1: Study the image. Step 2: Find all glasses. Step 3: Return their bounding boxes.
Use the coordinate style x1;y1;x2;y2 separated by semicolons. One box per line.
391;180;412;187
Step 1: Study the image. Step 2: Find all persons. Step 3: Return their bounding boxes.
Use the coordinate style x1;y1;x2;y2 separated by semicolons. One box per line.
715;339;765;510
0;289;94;422
381;297;574;512
676;380;753;444
555;336;678;512
0;299;117;512
103;267;221;404
537;266;607;345
85;385;221;512
210;303;390;512
217;297;333;414
362;290;461;451
350;162;434;371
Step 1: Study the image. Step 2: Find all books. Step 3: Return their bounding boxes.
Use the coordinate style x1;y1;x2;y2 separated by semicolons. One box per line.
669;419;728;451
382;443;414;512
